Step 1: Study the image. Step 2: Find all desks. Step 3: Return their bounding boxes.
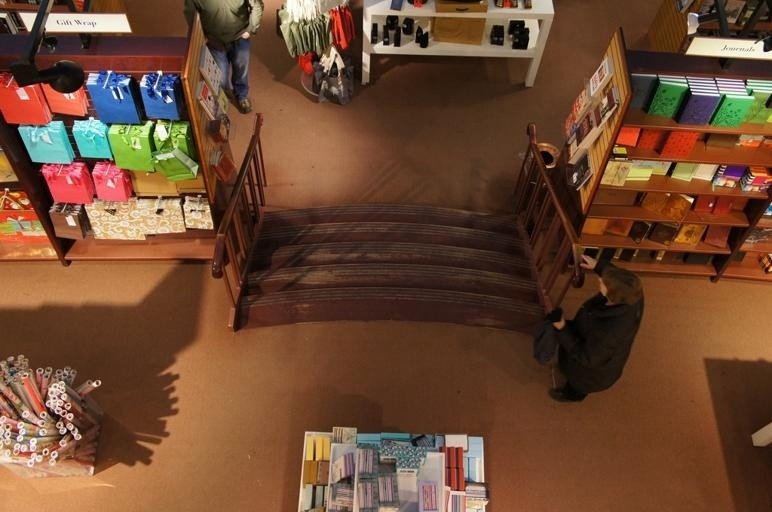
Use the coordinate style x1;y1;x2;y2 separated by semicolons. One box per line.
296;429;489;512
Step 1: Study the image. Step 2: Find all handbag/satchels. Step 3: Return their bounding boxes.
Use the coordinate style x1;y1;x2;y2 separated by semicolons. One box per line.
1;71;216;248
276;0;355;107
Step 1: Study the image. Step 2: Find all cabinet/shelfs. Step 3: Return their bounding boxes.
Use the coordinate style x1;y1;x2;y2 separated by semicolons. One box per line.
359;0;557;88
0;8;251;270
553;23;772;285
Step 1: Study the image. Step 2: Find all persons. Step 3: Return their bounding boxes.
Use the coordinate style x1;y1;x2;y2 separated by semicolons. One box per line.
547;253;645;402
183;0;264;114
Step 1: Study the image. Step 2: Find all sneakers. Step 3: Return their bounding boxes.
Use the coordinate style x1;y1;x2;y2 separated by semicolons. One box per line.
549;389;573;400
238;98;253;114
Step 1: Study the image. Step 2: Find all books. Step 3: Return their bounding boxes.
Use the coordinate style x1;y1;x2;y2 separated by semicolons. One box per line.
196;46;236;185
581;72;772;275
300;426;488;512
